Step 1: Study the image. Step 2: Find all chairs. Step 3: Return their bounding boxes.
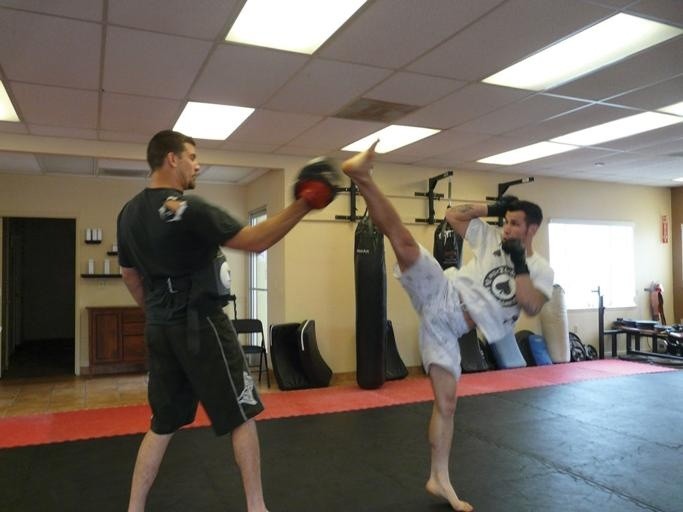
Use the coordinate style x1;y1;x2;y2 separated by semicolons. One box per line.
227;319;272;389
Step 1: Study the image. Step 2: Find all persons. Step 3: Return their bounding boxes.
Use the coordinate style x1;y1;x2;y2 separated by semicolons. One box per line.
114;128;341;512
338;135;558;512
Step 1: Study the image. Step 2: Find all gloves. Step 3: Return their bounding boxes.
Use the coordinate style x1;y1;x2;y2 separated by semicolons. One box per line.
488;194;518;217
296;161;340;210
501;238;528;275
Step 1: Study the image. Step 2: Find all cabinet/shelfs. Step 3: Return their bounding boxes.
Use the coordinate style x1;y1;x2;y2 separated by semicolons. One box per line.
80;239;122;279
84;303;152;378
